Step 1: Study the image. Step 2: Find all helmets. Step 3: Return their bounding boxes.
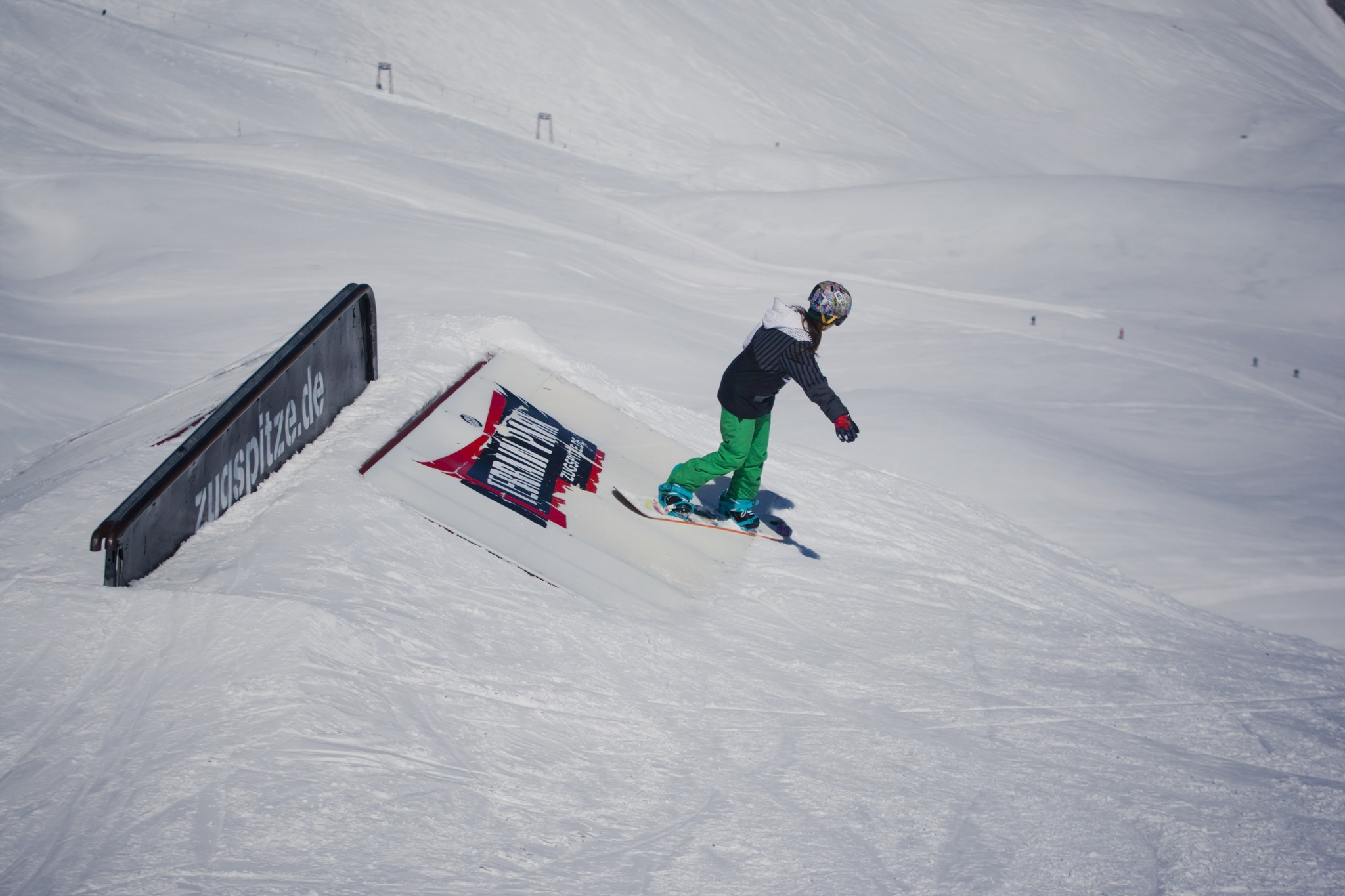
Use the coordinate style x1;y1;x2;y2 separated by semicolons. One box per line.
807;279;852;318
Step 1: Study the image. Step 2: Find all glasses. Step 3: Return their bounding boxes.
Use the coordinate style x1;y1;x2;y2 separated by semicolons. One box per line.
835;315;848;326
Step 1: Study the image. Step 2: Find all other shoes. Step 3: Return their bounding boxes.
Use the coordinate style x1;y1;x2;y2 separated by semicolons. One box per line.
721;493;760;530
665;492;691;515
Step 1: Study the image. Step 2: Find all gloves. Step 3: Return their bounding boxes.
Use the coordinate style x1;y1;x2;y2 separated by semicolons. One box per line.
833;413;859;443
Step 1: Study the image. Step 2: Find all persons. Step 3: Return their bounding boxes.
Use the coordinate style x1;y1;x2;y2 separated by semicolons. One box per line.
1253;357;1257;366
1119;330;1123;339
1294;369;1299;378
659;281;860;531
1032;317;1035;324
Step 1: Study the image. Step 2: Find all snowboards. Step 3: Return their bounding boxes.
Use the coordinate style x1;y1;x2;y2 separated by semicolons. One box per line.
613;484;792;541
